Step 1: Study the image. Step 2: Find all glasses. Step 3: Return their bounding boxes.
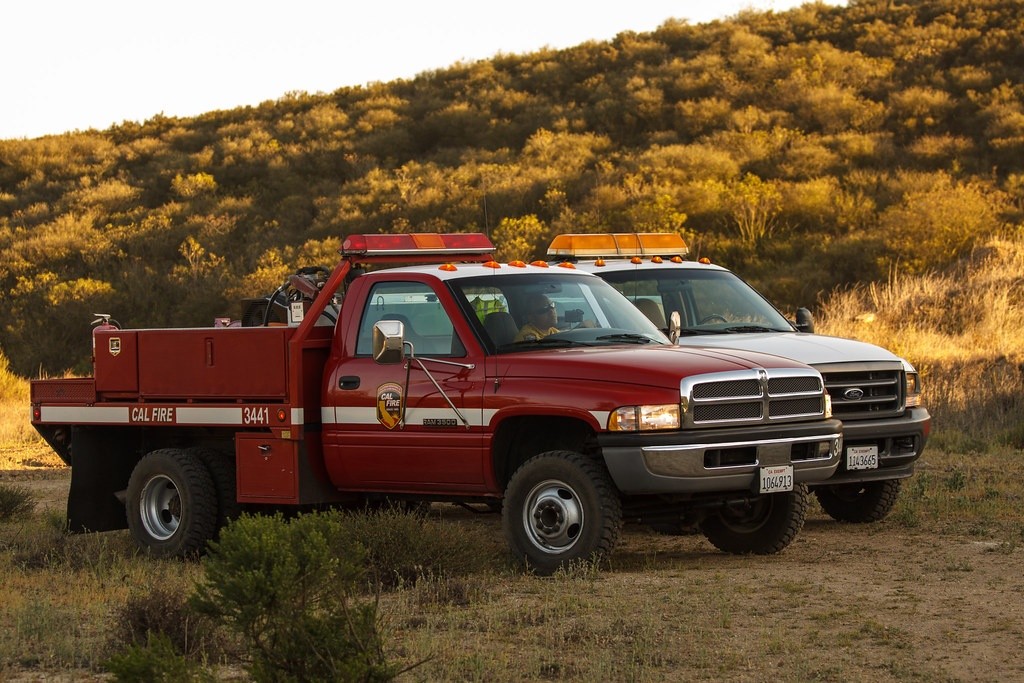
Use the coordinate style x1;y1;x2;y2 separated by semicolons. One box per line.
525;301;556;314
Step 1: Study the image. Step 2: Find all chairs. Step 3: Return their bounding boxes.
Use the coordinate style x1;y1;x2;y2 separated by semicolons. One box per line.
382;314;429;354
484;312;520;347
634;299;668;329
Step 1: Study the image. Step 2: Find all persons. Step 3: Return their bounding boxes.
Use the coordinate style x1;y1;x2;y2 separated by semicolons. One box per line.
513;295;595;343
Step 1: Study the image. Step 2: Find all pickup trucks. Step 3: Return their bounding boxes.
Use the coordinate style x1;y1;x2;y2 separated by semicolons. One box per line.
31;232;932;579
